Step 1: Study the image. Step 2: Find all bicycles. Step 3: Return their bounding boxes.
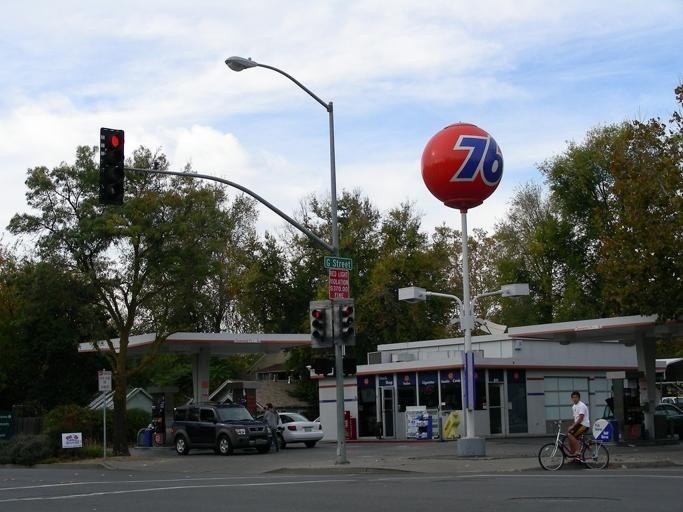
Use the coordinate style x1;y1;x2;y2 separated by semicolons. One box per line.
536;417;610;472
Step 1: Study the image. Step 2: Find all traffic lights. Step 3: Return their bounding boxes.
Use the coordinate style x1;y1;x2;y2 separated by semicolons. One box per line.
315;360;330;373
99;127;125;207
344;358;356;374
334;299;356;346
311;301;332;348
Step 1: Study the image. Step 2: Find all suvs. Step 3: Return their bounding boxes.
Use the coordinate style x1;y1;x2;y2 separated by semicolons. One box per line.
170;399;272;456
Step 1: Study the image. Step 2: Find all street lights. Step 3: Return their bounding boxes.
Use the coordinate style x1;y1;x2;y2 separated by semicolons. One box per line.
395;282;534;437
224;55;350;466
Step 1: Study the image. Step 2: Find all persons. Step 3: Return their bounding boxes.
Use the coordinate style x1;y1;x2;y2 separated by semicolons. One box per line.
263;403;280;453
567;391;590;462
444;410;460;439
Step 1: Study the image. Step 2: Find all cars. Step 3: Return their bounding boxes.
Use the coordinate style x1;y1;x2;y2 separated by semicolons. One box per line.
661;396;683;404
257;409;323;449
600;396;682;446
0;404;38;440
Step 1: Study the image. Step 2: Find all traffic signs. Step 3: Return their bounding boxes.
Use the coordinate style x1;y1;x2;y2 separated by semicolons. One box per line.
329;268;349;300
324;256;352;270
